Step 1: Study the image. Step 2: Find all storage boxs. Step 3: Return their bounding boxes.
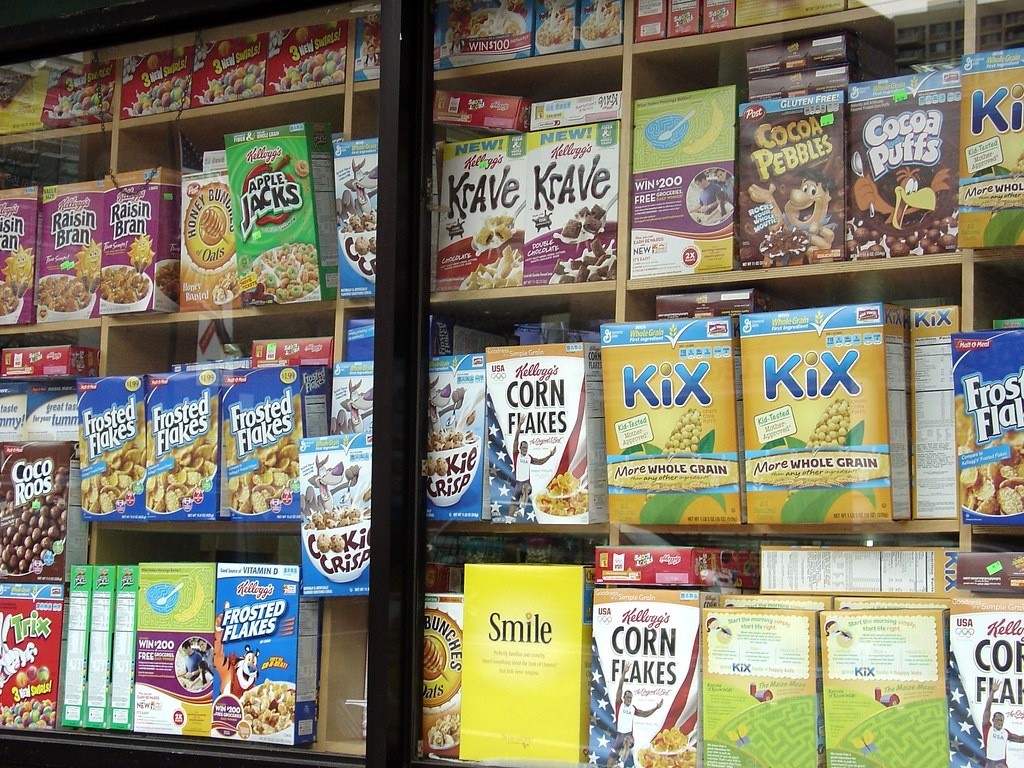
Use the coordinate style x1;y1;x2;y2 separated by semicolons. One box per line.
0;0;1024;768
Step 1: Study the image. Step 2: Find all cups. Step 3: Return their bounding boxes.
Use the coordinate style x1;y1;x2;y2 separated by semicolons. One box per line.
424;431;483;508
301;511;371;582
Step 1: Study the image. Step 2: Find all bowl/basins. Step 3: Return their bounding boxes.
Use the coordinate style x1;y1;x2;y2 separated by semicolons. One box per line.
531;487;589;526
338;220;378;284
153;257;182;312
443;6;619;66
633;746;697;768
36;274;96;323
99;265;153;313
1;280;24;324
253;244;321;303
236;680;295;747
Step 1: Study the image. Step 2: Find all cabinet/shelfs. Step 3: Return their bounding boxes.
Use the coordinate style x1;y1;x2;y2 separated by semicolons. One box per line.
0;0;1024;768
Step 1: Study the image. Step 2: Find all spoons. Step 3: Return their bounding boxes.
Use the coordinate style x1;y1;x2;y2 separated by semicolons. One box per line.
547;472;589;499
560;193;621;244
651;730;699;755
470;201;525;252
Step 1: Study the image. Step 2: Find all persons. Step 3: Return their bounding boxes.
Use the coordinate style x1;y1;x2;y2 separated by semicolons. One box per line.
177;638;213;691
688;169;734;224
982;678;1024;768
362;45;379;67
606;660;663;768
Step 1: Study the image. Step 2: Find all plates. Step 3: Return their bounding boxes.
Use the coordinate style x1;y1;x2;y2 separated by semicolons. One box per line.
211;274;242;306
